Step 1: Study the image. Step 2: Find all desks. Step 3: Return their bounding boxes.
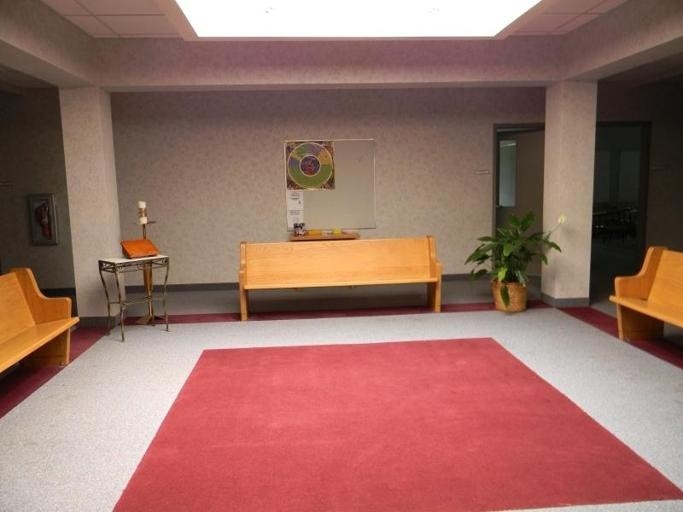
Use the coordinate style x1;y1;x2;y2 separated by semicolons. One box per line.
97;254;169;342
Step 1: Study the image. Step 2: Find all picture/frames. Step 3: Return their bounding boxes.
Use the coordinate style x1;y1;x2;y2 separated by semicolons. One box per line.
25;193;57;246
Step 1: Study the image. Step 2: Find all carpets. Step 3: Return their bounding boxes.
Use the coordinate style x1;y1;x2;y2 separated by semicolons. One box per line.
108;334;682;511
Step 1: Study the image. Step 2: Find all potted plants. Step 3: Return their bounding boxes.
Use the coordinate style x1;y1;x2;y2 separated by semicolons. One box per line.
462;210;566;313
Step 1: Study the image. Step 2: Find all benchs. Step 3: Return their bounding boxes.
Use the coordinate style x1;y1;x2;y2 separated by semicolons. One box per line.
237;235;441;322
0;266;80;374
607;244;682;343
